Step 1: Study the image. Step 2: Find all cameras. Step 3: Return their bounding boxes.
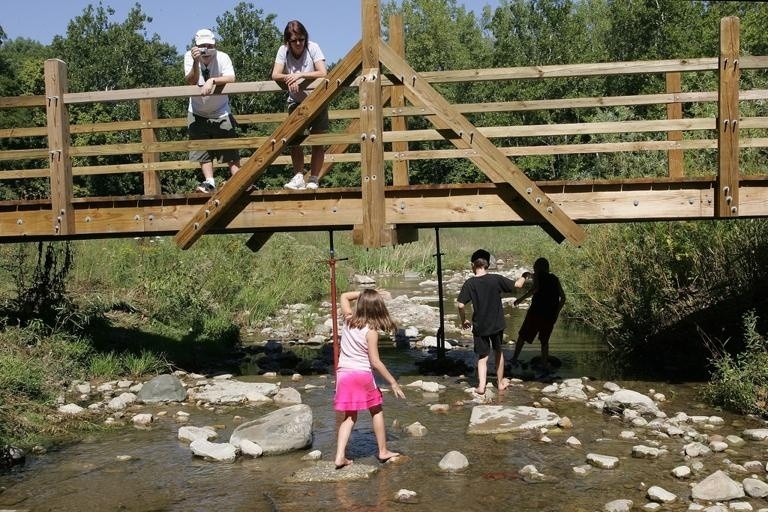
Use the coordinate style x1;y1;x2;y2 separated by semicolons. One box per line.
198;47;216;56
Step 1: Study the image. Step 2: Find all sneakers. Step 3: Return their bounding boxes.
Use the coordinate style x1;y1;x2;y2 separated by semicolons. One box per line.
307;176;318;190
196;181;216;193
284;171;306;190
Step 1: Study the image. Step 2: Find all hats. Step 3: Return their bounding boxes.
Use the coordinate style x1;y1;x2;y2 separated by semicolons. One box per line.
195;28;215;45
471;248;490;263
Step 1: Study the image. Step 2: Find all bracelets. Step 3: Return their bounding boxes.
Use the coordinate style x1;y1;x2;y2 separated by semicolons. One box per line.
211;77;216;85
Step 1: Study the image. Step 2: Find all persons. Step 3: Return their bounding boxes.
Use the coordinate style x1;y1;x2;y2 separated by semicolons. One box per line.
456;249;531;395
332;286;407;467
183;29;256;195
507;258;565;367
271;20;327;190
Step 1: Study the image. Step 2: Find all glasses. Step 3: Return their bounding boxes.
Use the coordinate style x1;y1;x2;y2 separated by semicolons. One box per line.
289;36;305;42
